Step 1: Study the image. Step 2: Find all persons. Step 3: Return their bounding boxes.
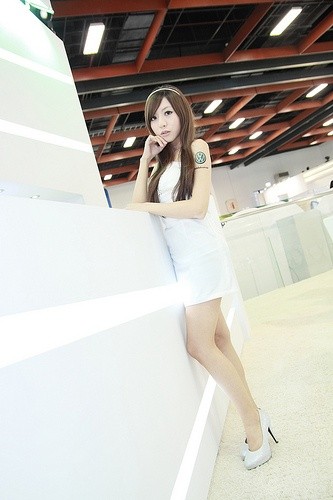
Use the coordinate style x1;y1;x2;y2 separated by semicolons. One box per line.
128;85;279;470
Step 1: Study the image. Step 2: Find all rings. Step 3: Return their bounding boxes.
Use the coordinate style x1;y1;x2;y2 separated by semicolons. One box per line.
147;135;153;142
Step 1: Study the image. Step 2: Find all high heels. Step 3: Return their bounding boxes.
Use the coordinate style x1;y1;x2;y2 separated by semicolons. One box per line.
244;410;278;470
240;408;261;457
